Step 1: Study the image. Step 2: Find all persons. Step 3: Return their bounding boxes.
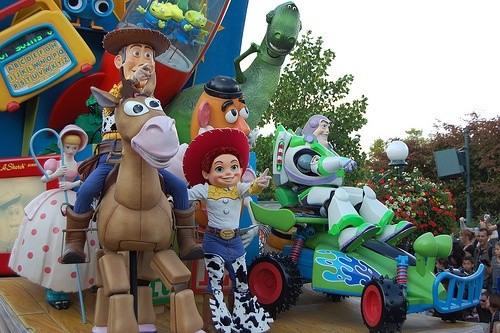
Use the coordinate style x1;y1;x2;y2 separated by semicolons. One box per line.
62;24;206;262
286;115;416;253
189;77;251;237
170;128;274;333
8;124;102;309
435;213;500;333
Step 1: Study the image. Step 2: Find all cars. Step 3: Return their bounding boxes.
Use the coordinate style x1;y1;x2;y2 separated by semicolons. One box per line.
245;200;486;333
